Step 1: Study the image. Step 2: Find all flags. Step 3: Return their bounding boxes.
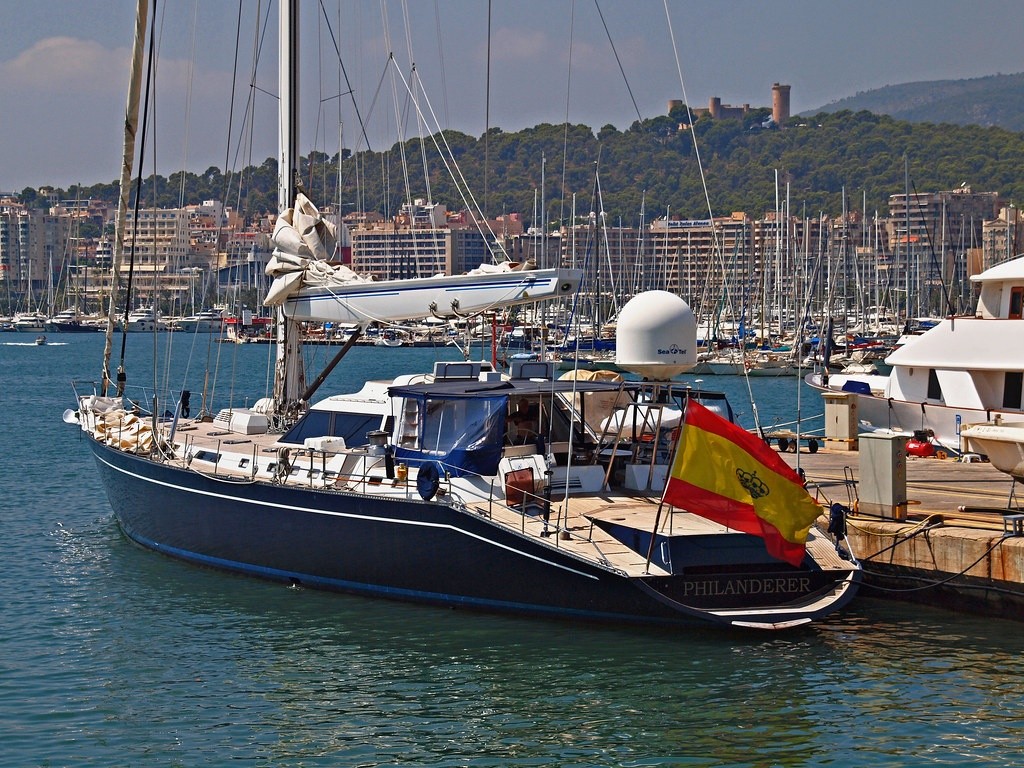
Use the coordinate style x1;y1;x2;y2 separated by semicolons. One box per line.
660;401;822;567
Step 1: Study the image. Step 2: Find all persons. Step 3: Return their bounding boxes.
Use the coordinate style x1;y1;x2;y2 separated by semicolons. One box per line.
505;399;540;443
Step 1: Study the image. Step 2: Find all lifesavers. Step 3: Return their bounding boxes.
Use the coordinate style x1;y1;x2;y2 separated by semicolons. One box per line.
416;461;439;501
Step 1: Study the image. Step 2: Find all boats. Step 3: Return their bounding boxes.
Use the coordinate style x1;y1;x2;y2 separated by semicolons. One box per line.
959;416;1023;487
807;254;1023;453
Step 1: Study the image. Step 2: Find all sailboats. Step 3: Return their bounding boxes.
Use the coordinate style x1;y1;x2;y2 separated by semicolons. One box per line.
2;156;1024;379
61;0;863;644
